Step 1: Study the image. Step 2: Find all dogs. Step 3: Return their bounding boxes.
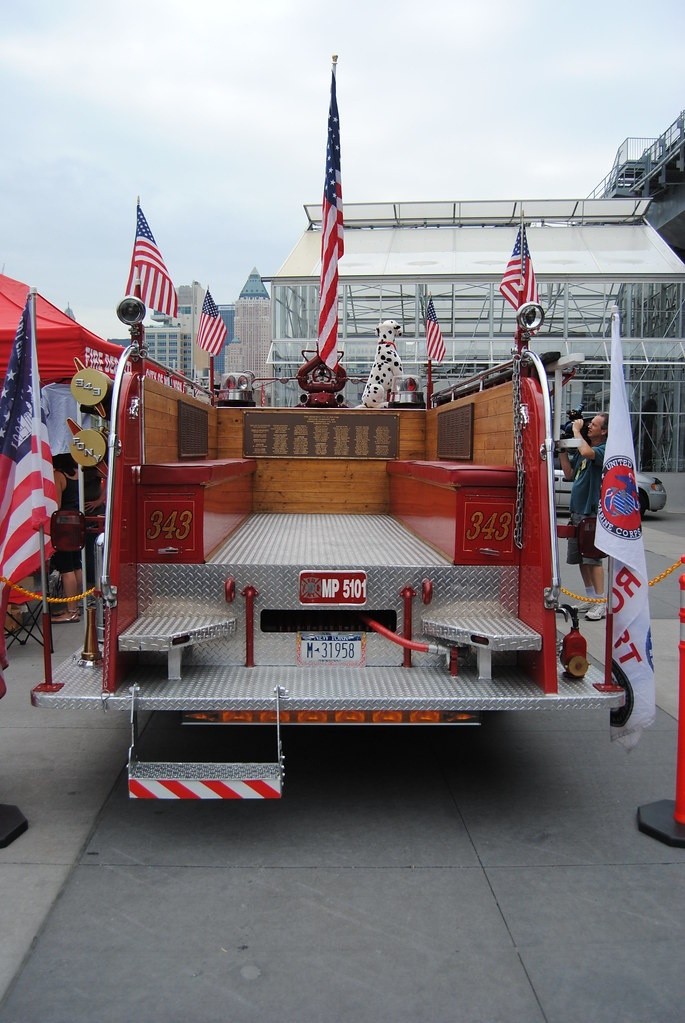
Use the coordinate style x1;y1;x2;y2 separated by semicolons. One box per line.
353;319;405;409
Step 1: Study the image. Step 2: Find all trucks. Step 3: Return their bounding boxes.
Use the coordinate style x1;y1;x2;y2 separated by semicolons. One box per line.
28;277;629;802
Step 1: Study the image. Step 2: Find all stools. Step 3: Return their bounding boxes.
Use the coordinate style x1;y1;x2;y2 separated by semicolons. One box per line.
5;586;55;654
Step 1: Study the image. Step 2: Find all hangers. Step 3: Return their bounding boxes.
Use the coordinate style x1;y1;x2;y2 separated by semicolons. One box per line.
55;377;73;383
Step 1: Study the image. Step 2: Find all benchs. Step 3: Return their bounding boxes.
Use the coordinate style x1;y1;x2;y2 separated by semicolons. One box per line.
138;457;257;564
386;460;518;565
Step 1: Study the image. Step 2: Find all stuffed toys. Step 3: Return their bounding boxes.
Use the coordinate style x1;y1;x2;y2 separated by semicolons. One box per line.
354;319;403;408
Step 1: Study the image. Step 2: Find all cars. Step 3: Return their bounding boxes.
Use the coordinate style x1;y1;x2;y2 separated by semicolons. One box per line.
553;448;669;521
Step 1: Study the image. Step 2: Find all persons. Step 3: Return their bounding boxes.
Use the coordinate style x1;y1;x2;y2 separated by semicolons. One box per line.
558;413;607;621
49;450;108;623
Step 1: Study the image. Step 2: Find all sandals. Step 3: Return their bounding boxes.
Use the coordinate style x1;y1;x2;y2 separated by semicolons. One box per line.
50;607;81;623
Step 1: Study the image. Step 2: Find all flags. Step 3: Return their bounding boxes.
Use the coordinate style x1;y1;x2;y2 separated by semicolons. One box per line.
318;69;345;376
0;296;59;591
499;223;541;337
125;205;178;319
593;315;656;756
426;298;447;363
196;289;229;356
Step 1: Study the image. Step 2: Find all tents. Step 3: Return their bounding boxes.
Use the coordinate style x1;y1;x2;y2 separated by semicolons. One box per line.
0;274;212;692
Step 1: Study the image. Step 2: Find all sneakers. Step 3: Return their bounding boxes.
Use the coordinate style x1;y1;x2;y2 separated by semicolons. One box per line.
585;603;606;620
571;601;595;612
